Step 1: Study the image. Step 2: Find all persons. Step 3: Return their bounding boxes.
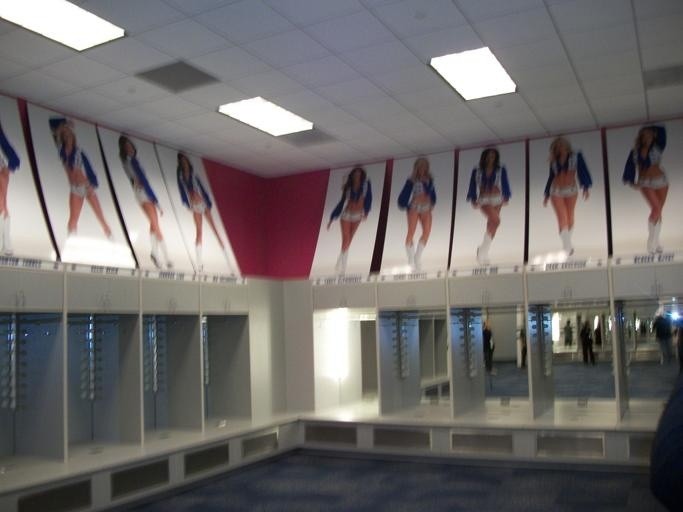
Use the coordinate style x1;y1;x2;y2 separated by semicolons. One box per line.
648;312;675;365
619;126;670;254
463;146;513;266
327;165;372;274
580;321;595;367
520;321;527;369
175;150;236;279
1;120;21;261
395;158;437;266
117;133;174;267
672;311;683;367
540;137;592;257
563;320;573;348
480;320;495;371
47;116;115;242
647;374;683;510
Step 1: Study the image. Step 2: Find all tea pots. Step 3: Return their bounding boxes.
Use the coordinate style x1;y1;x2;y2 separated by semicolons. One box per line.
0;311;251;464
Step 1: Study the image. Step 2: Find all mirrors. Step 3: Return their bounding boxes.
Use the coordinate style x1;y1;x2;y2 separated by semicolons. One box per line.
339;296;682;417
0;311;251;464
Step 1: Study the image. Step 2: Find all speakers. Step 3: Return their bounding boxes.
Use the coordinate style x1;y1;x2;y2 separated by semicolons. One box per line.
477;234;494;266
195;244;204;272
560;226;576;256
0;211;13;258
148;234;176;272
223;247;238;276
336;249;349;275
407;241;426;270
647;218;664;255
